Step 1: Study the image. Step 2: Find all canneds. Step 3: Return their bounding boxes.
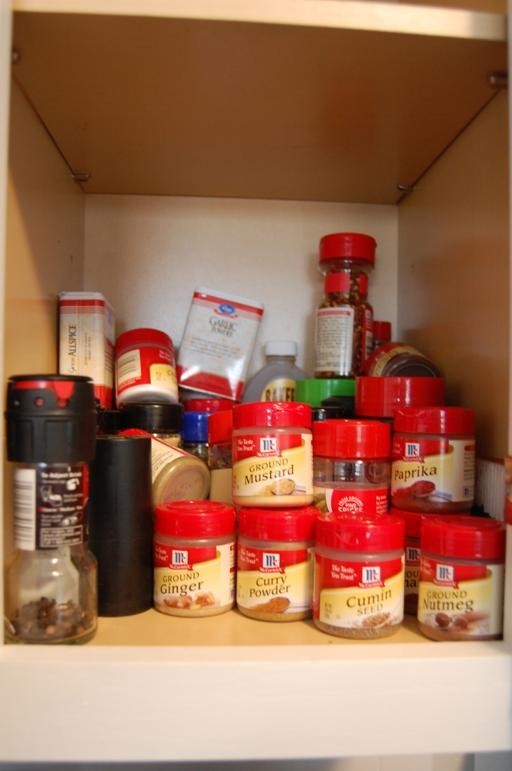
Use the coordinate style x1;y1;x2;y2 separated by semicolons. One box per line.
416;512;504;641
311;419;392;516
311;508;404;640
149;500;239;618
231;400;315;506
118;428;209;504
392;509;440;616
353;376;444;436
364;340;441;374
112;327;179;404
293;376;356;421
186;396;237;470
388;403;478;515
234;504;320;621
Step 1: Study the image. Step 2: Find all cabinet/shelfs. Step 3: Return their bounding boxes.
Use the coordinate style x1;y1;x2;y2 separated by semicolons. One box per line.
0;0;511;768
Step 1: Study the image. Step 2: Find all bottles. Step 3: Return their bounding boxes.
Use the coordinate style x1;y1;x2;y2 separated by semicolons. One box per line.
6;370;99;643
242;342;305;404
